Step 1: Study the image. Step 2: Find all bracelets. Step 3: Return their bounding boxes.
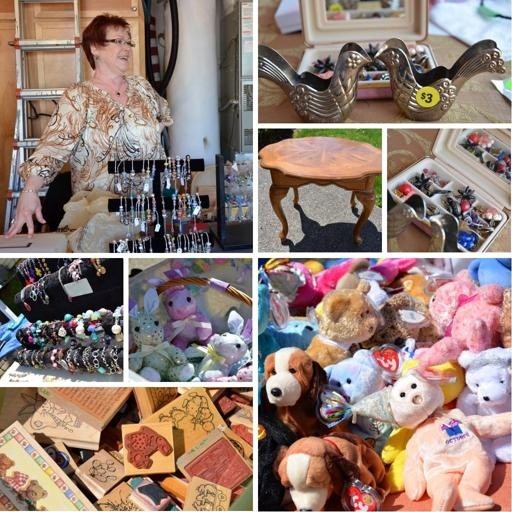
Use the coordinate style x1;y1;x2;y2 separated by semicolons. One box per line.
17;258;123;376
111;154;211;253
21;189;37;193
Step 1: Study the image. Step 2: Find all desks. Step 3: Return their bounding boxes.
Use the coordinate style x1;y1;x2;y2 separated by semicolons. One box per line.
0;232;70;253
259;137;382;245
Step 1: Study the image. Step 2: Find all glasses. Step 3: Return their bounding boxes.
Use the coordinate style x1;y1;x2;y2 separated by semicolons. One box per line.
105;38;136;48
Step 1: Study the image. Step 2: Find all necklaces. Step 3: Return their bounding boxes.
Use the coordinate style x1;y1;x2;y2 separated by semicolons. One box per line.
93;76;125;97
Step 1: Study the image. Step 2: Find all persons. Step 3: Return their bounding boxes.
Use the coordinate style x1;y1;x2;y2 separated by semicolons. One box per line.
4;13;174;253
129;284;252;383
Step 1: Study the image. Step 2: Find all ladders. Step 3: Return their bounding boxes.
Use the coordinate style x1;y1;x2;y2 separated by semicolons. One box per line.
4;0;81;236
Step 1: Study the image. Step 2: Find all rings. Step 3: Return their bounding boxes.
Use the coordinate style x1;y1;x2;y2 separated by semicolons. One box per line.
11;218;14;224
